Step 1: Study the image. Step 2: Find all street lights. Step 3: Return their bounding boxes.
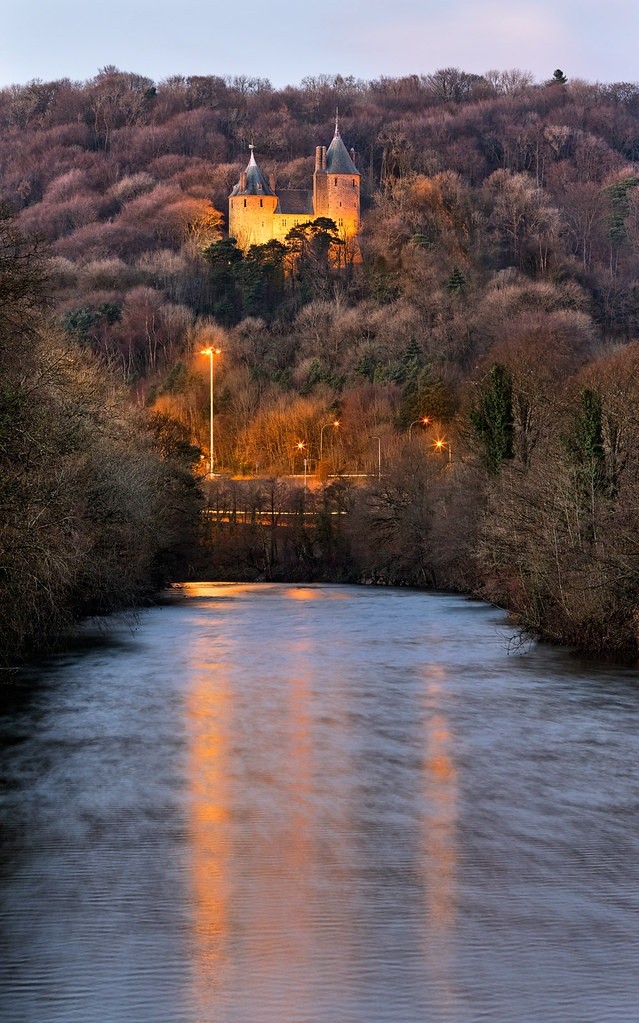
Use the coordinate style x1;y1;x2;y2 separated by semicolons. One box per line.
321;422;338;464
200;346;221;474
437;442;451;463
408;419;428;443
369;435;381;482
